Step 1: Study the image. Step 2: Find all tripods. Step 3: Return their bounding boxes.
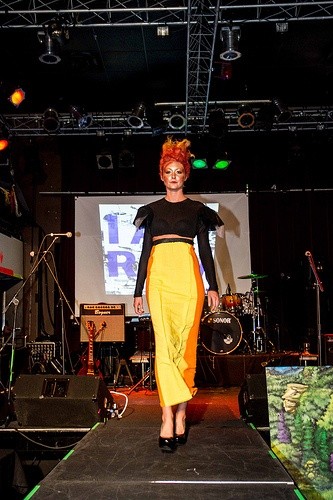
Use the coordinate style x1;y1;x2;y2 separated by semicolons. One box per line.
241;278;277;355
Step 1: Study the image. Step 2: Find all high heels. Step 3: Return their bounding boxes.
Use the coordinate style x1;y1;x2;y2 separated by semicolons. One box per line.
159;431;174;452
174;424;186;446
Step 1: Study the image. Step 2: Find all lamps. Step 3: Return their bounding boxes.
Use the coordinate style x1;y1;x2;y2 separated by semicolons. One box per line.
43;104;256;171
156;24;170;36
276;20;289;34
220;26;241;62
37;28;70;65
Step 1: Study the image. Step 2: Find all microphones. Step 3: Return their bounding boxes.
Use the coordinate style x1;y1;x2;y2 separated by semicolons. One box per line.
47;232;72;237
29;251;50;256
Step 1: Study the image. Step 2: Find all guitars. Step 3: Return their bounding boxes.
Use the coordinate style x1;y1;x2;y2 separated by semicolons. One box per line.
76;321;103;379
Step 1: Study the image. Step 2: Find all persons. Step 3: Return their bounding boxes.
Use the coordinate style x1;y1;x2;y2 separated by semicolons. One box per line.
32;357;48;375
133;138;225;451
78;343;113;416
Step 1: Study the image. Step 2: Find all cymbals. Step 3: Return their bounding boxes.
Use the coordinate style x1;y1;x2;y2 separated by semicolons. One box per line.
237;272;271;279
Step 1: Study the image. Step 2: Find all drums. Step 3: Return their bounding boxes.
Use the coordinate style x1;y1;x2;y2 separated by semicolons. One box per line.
136;316;155;356
222;293;243;314
200;311;243;355
202;294;222;316
241;295;266;315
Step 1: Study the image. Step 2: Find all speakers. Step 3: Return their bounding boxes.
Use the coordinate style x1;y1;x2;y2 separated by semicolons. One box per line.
12;374;111;428
237;374;268;418
80;304;126;342
